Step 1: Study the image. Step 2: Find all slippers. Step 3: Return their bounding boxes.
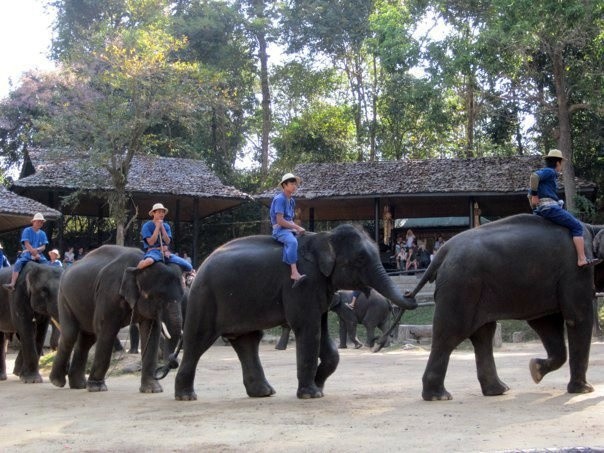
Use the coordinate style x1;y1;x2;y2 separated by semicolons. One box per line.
292;273;307;289
580;257;603;270
344;301;355;310
2;283;16;292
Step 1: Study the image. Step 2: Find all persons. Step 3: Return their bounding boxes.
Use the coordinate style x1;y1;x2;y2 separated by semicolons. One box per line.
346;290;360;311
269;173;307;290
394;230;446;270
2;212;48;290
64;246;75;262
76;247;84;259
125;203;196;277
0;242;11;268
45;248;63;267
528;149;602;269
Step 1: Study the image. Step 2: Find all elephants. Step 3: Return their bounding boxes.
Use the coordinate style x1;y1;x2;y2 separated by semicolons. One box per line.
153;223;418;401
0;261;63;384
371;214;604;401
275;323;291;350
329;289;399;349
49;245;186;393
50;324;139;354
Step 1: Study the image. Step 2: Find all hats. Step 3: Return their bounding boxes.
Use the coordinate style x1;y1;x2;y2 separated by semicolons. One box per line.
541;148;568;161
48;249;61;258
31;213;47;223
277;173;301;188
148;202;169;217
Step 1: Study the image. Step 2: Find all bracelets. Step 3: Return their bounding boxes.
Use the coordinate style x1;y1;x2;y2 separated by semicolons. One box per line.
530;189;539;197
